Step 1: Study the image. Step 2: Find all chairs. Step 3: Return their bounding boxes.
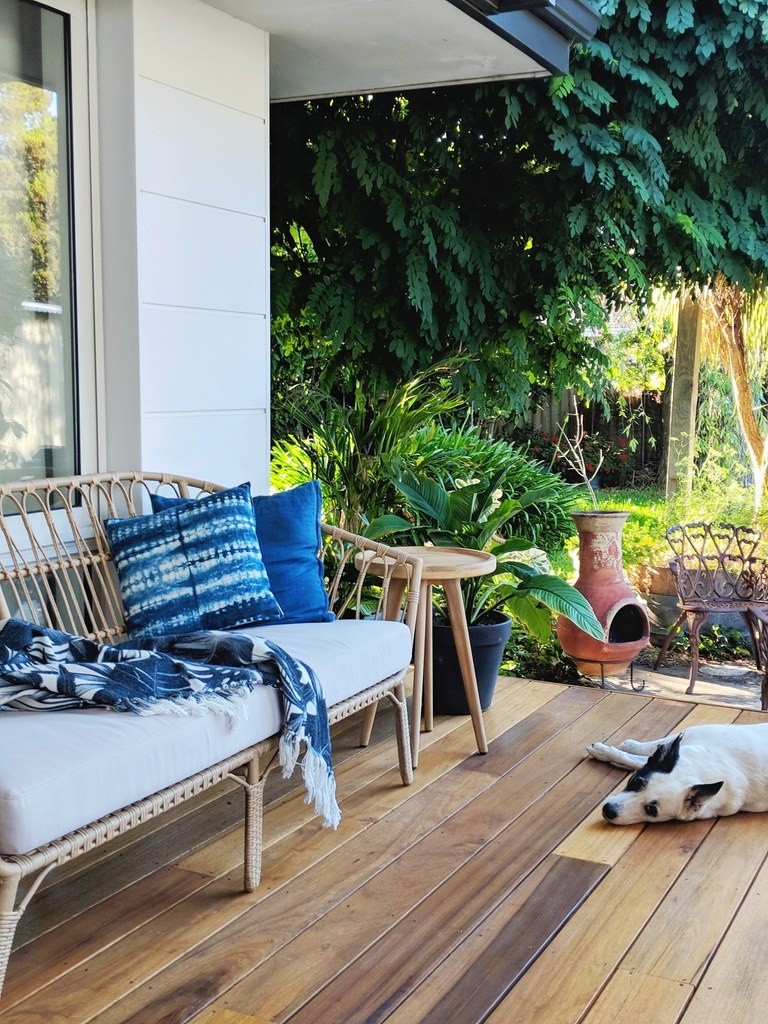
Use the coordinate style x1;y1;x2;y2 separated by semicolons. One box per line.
653;521;768;711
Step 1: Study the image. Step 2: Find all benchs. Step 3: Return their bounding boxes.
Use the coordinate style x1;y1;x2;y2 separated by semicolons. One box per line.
0;471;425;992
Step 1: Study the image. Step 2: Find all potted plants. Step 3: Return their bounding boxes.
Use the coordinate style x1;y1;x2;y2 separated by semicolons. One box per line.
364;460;607;714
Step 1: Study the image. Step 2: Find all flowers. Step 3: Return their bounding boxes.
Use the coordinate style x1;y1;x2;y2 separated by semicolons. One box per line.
563;431;635;476
525;430;559;462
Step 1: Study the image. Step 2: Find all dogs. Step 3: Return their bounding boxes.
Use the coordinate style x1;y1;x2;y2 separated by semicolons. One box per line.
586;722;768;825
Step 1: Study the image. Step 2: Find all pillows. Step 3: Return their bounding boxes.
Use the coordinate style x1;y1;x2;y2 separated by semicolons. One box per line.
102;481;285;641
150;479;337;631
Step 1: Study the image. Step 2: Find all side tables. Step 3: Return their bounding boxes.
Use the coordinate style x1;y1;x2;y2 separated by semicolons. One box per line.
354;545;497;770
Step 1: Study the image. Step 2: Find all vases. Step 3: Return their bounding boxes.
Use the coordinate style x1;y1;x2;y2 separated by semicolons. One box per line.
579;471;604;489
539;465;556;473
